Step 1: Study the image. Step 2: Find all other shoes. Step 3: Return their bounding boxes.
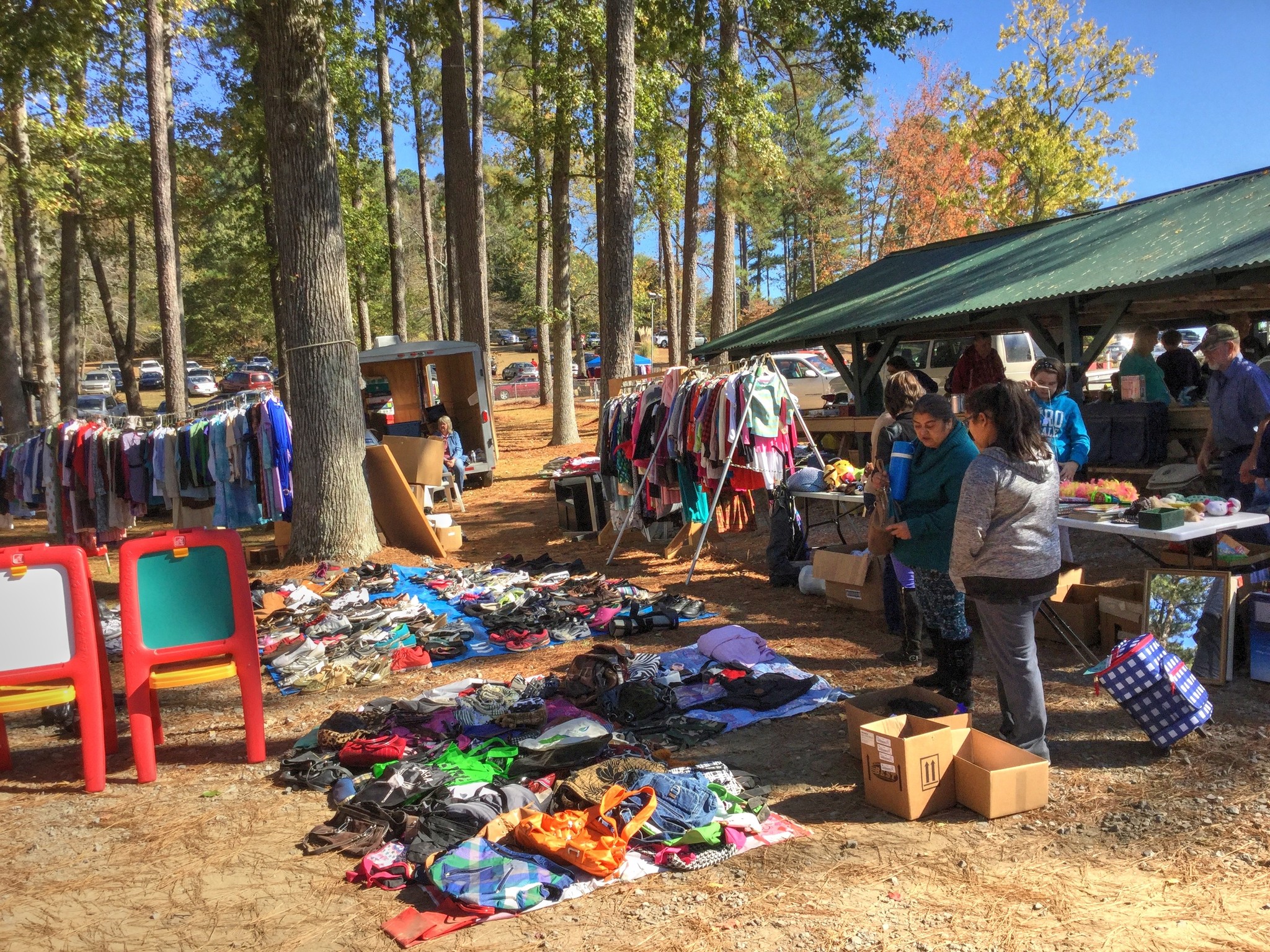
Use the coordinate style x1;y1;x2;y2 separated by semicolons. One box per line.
250;551;707;729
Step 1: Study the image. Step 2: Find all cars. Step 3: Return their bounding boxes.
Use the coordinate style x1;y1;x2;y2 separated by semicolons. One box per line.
538;353;599;377
491;358;540;400
79;355;279;420
653;330;668;348
770;345;856;410
519;328;538;352
695;331;708;347
1105;327;1208;363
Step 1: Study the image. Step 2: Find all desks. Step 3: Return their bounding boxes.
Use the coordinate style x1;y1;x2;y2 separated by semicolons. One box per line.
790;489;1270;573
793;406;1213;468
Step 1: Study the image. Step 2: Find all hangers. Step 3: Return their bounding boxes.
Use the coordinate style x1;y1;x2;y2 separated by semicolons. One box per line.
619;355;768;396
0;382;280;454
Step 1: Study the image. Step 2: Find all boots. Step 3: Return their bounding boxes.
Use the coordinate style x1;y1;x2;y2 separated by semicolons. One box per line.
913;626;948;689
939;636;976;706
883;586;924;668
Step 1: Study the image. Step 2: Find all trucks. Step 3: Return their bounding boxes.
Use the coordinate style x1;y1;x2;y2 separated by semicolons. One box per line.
360;340;497;488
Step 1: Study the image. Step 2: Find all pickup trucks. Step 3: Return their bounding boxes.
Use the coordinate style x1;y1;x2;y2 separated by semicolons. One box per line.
76;395;127;429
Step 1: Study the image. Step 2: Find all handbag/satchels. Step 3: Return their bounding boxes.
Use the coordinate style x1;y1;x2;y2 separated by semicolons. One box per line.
278;729;772;917
867;459;900;555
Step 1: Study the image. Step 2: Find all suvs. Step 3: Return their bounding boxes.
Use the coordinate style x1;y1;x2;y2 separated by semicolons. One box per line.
572;332;600;350
492;329;519;346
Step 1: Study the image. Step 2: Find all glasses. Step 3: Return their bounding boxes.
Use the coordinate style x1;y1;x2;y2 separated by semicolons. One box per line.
962;413;991;428
1240;323;1254;327
1201;341;1229;356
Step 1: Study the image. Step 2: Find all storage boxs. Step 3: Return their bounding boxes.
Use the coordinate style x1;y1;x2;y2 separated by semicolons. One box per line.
1138;507;1184;530
381;435;445;487
813;541;887;612
246;520;293;568
1032;583;1100;648
1120;374;1147;403
1248;591;1270;682
1204;533;1250;559
1099;583;1146;648
844;684;978;762
859;714;957;821
951;727;1049;819
372;485;462;552
363;376;448;437
1159;533;1270;641
1050;559;1087;601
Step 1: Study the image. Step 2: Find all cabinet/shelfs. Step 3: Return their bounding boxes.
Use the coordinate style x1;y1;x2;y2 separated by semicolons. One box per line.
554;475;607;532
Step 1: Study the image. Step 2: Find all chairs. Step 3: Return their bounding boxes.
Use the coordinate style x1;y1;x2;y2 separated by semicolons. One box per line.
119;525;266;784
427;472;467;513
74;532;113;576
0;543;122;792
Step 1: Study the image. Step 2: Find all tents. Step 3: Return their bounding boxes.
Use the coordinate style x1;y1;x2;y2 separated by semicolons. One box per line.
586;351;651;368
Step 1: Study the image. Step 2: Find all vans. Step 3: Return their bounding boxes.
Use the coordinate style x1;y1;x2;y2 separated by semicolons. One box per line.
863;330;1046;396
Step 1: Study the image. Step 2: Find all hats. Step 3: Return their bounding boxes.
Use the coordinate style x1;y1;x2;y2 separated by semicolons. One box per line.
1192;323;1239;352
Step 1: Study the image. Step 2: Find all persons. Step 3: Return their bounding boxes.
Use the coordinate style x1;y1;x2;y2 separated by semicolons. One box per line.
1191;323;1270;544
1229;315;1269;365
577;373;588;383
531;358;537;367
1017;357;1091;570
1111;371;1120;390
859;342;886;462
1251;410;1270;492
864;404;908;634
1155;328;1202;402
872;371;936;670
432;416;465;502
1120;326;1177;408
889;356;938;395
889;394;980;712
1059;344;1090;392
949;377;1061;765
1190;577;1223;680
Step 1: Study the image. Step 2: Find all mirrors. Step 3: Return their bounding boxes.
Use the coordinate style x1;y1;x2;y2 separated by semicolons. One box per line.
1141;567;1226;686
